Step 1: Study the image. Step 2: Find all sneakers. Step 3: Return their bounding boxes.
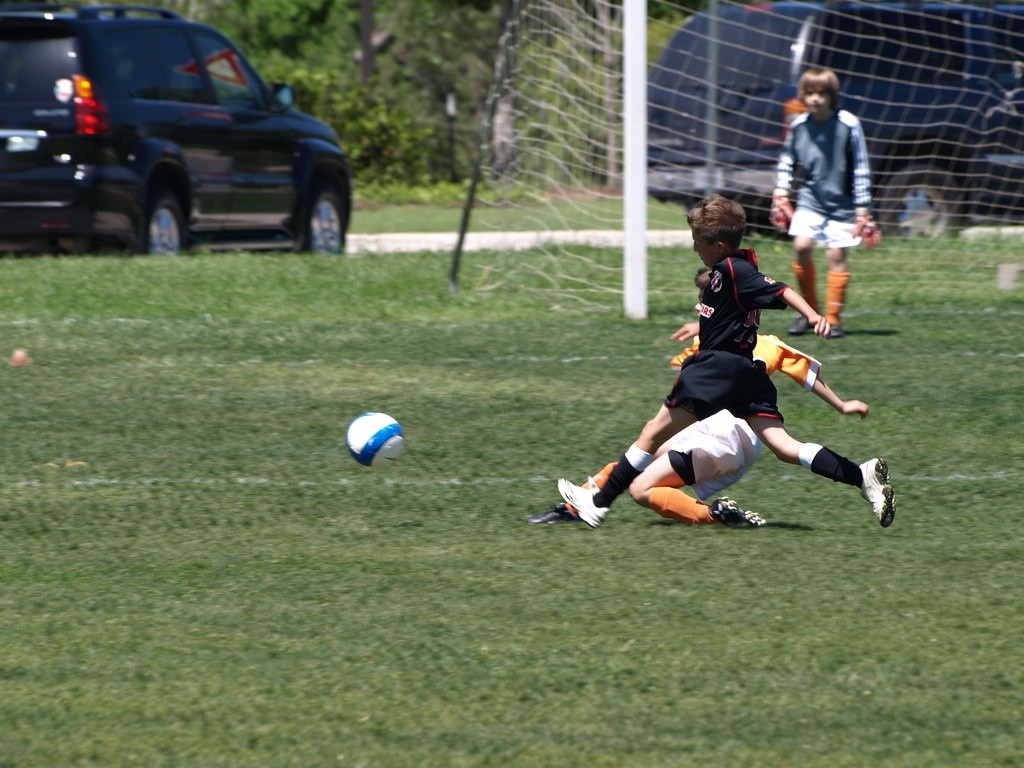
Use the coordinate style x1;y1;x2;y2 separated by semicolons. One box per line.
708;496;766;528
528;502;574;524
557;474;610;529
858;456;896;528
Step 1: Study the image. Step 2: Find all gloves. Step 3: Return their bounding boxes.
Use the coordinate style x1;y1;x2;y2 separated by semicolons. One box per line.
769;196;794;232
851;209;881;247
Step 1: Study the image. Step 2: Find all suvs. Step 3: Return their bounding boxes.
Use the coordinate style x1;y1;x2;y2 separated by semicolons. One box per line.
642;1;1024;247
0;1;357;255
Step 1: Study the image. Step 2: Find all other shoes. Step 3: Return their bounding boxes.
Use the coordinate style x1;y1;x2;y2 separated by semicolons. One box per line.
788;316;813;335
829;326;844;337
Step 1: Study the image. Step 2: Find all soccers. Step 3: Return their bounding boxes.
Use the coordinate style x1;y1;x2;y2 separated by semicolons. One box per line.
346;412;404;467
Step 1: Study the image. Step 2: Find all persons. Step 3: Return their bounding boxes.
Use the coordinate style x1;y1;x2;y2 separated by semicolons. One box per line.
528;195;896;531
770;68;878;338
